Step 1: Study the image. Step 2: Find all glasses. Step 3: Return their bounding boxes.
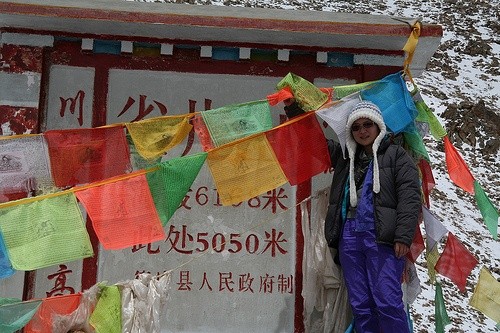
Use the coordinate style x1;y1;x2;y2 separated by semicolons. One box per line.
351;120;375;131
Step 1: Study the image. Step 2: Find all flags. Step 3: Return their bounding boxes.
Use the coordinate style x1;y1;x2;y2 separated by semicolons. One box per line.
0;19;500;333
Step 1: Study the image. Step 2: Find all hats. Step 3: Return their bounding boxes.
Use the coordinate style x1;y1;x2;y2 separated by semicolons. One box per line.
346;101;385;207
